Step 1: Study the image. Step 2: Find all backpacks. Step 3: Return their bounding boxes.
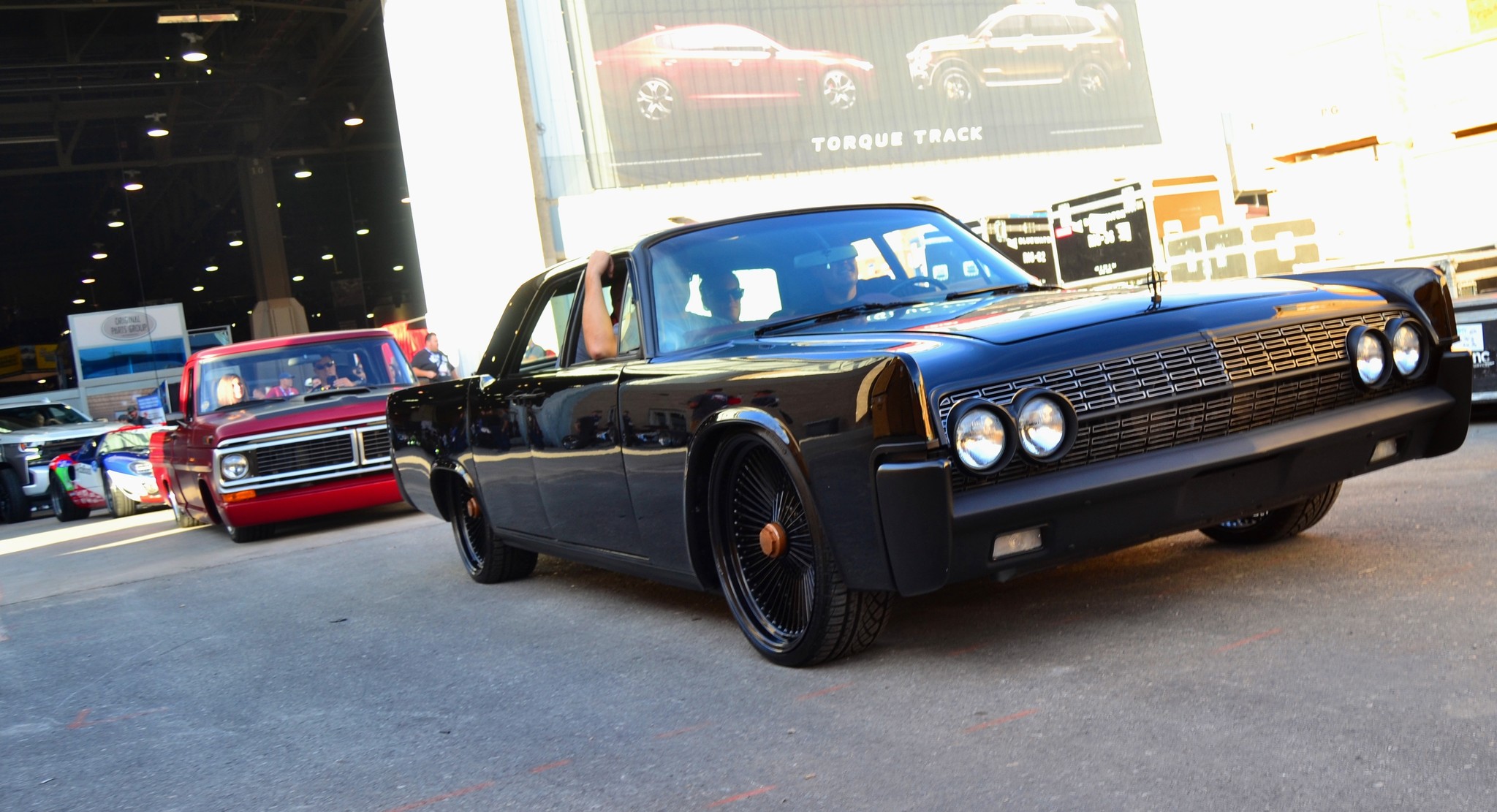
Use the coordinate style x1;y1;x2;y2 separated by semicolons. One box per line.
529;345;556;356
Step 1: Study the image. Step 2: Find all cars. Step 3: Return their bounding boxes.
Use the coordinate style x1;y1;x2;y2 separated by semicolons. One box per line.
593;20;876;125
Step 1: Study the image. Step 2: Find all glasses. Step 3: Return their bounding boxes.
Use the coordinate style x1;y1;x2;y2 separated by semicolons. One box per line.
709;288;744;303
316;360;335;370
660;271;693;284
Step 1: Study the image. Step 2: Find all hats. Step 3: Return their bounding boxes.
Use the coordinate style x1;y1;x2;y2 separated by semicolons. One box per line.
305;377;312;387
127;406;135;415
279;373;294;379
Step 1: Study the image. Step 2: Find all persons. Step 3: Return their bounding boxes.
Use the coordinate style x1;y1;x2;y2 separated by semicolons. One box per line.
698;267;744;323
310;376;322;390
309;353;363;395
217;373;255;408
126;406;152;425
28;413;63;428
551;275;624;368
264;371;299;400
580;246;733;361
521;335;546;365
117;414;135;425
410;332;460;385
768;238;944;322
304;378;312;393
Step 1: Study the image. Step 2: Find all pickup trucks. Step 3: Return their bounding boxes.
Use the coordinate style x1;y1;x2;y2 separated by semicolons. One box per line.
148;328;421;544
384;198;1475;671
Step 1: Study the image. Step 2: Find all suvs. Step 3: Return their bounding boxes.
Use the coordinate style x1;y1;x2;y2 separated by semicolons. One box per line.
907;2;1131;108
0;396;135;526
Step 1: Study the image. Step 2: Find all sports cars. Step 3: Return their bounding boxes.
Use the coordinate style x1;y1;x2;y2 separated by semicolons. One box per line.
49;420;181;522
